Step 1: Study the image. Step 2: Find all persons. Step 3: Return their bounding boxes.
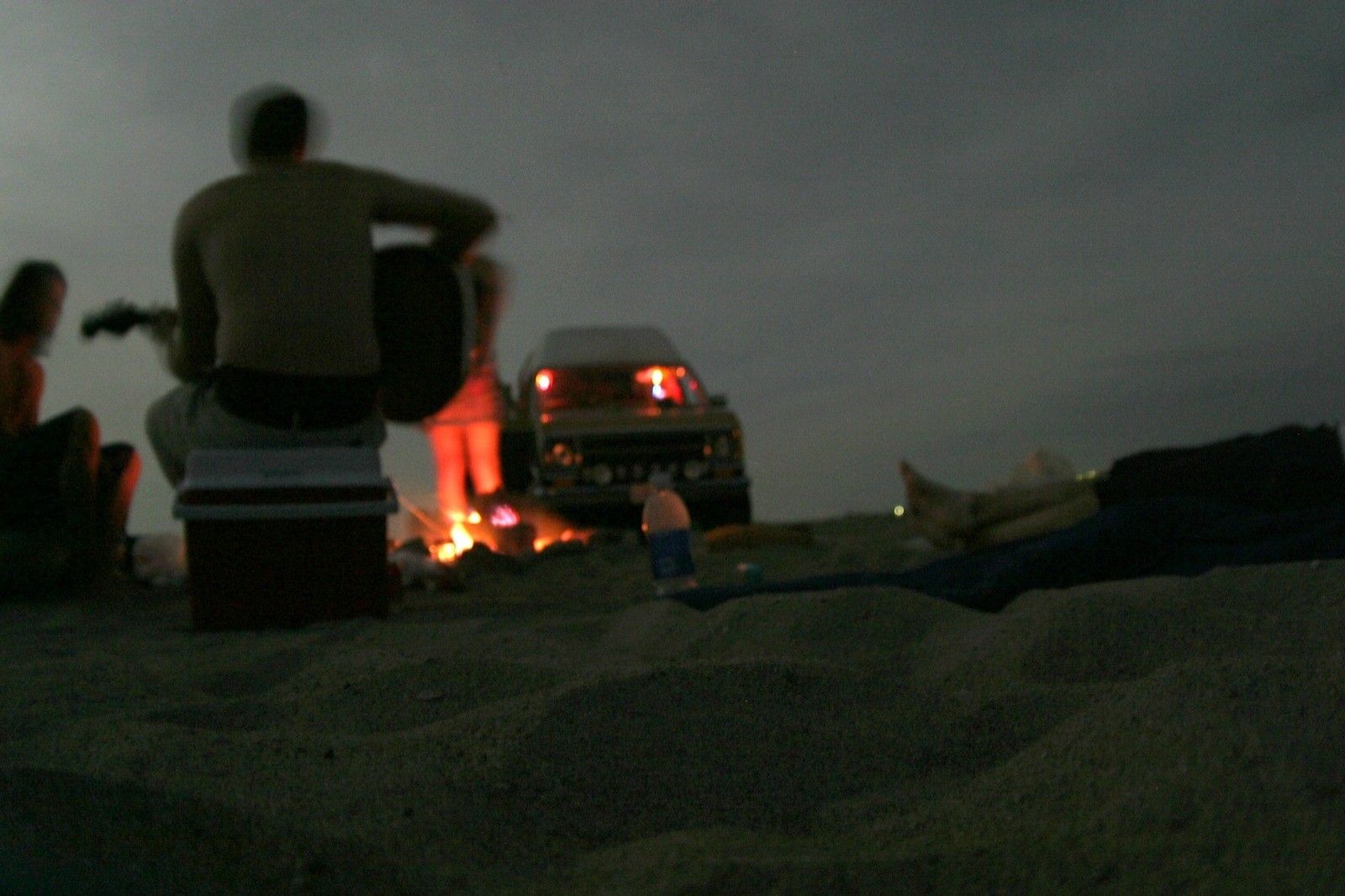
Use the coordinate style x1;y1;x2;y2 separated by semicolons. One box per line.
145;86;509;553
0;261;150;597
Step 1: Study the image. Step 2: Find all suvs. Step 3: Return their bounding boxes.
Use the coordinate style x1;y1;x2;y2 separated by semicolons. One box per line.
517;323;752;530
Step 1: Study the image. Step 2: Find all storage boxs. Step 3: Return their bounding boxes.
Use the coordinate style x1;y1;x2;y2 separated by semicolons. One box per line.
171;444;399;629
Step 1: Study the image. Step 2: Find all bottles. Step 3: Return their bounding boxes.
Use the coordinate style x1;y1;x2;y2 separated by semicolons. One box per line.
642;477;696;592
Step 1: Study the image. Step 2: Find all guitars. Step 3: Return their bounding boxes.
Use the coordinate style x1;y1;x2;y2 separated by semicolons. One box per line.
87;239;498;424
